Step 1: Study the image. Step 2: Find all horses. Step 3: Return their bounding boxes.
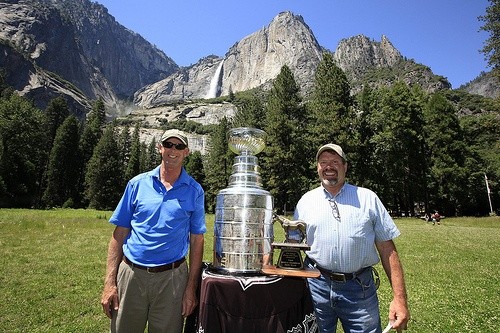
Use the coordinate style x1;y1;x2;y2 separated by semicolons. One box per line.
271;209;308;244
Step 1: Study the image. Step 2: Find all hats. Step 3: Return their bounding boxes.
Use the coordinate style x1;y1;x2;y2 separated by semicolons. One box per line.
160;129;188;149
316;143;348;162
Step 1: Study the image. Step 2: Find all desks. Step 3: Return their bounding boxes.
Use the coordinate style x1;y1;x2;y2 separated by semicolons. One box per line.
184;267;319;333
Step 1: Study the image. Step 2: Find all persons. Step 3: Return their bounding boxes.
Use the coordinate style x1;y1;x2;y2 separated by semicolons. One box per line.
100;129;207;333
425;212;440;225
288;143;410;333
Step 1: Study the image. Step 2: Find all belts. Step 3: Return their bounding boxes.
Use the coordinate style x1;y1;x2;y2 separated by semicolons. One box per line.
304;255;372;281
123;254;187;272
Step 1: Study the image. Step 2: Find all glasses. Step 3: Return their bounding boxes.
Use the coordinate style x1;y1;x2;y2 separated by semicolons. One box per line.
161;141;187;150
329;198;340;219
